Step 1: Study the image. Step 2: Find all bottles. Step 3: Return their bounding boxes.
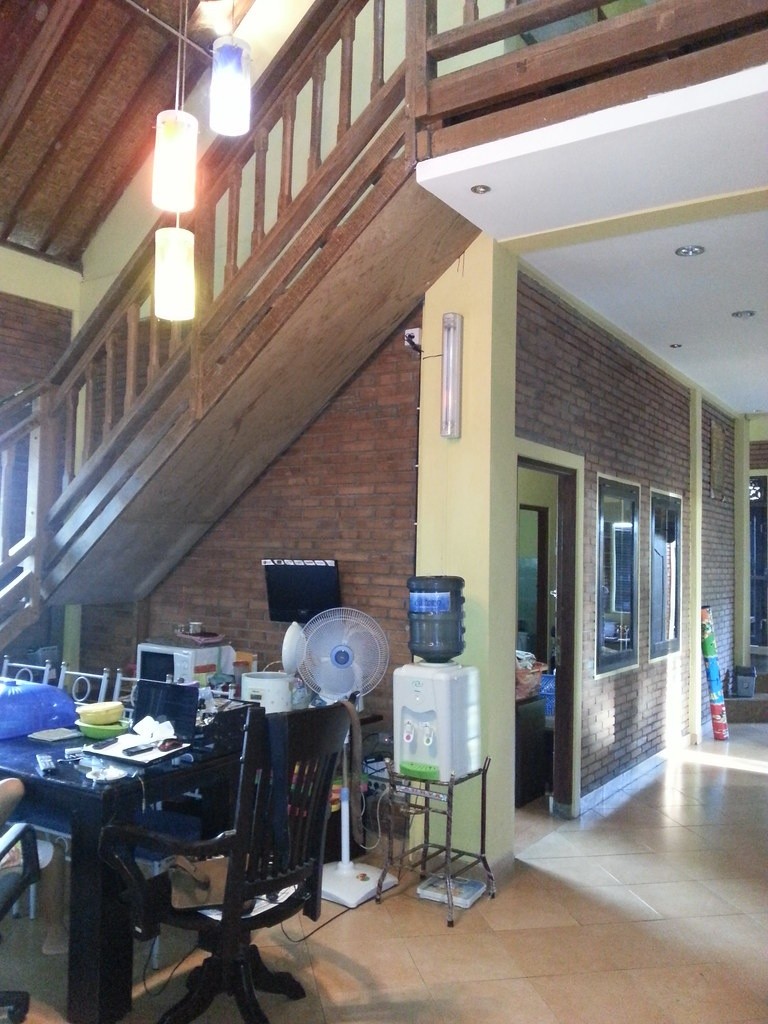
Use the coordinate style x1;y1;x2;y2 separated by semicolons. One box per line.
407;575;468;663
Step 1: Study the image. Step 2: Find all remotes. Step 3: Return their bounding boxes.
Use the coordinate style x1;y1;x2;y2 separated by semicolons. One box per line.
36;755;56;773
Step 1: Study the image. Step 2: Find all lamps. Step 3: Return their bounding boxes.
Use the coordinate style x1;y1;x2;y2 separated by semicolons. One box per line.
149;0;198;214
208;0;251;138
154;0;197;321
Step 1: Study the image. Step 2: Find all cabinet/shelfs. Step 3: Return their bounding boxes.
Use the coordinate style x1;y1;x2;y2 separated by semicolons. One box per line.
375;756;498;928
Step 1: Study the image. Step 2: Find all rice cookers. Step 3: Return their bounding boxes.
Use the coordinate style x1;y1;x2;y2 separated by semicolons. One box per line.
241;620;307;712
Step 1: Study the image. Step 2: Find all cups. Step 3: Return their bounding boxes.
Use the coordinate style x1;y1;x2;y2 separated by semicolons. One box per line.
189;621;204;634
233;661;249;697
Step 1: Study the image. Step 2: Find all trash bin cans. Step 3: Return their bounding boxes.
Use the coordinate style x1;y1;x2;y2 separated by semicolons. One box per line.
735;665;758;697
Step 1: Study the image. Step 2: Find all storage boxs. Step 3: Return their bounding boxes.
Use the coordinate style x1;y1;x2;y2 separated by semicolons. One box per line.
537;674;556;717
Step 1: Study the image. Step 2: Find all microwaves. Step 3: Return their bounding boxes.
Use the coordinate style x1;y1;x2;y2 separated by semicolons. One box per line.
136;642;231;688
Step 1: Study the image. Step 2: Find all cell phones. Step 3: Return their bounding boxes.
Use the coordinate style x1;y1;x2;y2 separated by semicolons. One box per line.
93;737;117;749
123;744;153;756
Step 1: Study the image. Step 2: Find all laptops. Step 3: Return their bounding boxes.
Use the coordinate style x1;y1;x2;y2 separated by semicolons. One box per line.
81;680;199;767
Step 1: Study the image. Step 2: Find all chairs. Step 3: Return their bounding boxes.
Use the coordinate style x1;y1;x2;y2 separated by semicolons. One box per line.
98;689;362;1023
57;660;111;708
0;655;51;685
111;667;174;721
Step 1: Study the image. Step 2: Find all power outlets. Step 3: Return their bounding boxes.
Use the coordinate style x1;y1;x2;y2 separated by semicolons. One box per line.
404;328;422;346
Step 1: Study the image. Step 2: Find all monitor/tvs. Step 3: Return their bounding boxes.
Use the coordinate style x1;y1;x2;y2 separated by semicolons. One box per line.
263;558;342;624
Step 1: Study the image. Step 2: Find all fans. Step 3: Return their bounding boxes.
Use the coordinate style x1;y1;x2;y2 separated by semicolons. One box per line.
293;607;399;911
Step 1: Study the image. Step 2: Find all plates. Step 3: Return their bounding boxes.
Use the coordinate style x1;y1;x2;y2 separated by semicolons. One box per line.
85;768;128;784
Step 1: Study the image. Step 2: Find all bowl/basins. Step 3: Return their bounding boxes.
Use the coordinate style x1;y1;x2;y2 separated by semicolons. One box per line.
75;701;124;725
74;719;130;740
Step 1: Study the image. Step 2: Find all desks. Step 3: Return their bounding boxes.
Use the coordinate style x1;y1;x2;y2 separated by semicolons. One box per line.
0;709;265;1024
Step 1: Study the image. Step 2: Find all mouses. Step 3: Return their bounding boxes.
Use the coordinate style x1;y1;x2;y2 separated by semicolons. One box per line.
159;738;182;752
